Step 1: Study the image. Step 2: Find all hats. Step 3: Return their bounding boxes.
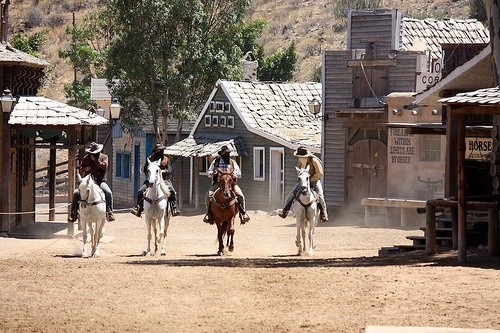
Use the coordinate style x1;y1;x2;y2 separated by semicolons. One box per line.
152;143;165;152
293;145;311;158
218;146;231;155
86;142;104;154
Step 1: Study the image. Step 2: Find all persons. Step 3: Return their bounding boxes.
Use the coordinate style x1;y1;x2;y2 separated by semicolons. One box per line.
279;146;328;223
131;143;181;217
203;146;250;223
68;142;115;222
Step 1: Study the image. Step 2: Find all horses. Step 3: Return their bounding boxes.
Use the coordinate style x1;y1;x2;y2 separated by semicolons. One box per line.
208;168;239;258
142;155;172;257
76;170;106;259
292;164;321;257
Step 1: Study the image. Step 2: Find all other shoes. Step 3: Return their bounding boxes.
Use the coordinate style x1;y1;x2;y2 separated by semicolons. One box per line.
242;214;248;221
320;212;328;222
173;206;180;215
69;212;78;221
106;211;115;220
280;208;290;218
205;214;211;221
134;206;144;211
